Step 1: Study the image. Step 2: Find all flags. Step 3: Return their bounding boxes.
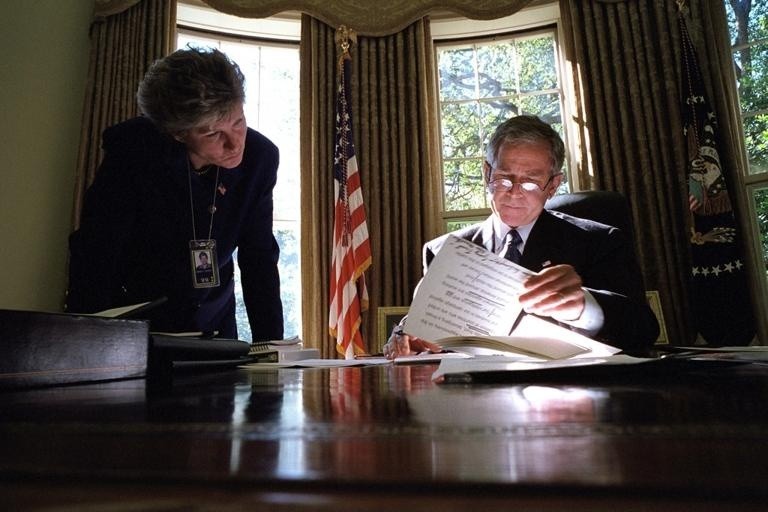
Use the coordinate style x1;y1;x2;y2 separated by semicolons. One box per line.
328;61;372;360
678;10;760;348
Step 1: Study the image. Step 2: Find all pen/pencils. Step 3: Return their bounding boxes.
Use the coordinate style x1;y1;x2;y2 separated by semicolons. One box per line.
507;261;555;336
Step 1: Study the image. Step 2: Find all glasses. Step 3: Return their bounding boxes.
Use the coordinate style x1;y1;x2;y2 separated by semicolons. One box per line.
485;161;563;191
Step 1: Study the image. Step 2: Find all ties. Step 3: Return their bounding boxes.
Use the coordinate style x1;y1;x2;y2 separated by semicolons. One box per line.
498;230;522;264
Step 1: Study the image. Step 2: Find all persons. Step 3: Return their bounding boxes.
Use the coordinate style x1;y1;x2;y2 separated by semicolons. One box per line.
66;48;283;341
197;252;212;283
382;116;661;360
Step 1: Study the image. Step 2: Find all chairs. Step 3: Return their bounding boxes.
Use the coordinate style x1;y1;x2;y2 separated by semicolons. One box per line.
422;189;648;356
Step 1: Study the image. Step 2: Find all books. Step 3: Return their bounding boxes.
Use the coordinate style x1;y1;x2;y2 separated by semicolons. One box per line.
249;336;320;363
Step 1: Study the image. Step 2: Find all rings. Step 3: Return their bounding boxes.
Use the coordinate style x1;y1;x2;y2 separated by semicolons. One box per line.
556;293;567;304
393;326;407;336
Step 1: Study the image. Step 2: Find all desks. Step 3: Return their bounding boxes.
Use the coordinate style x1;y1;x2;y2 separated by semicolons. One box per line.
1;359;768;506
